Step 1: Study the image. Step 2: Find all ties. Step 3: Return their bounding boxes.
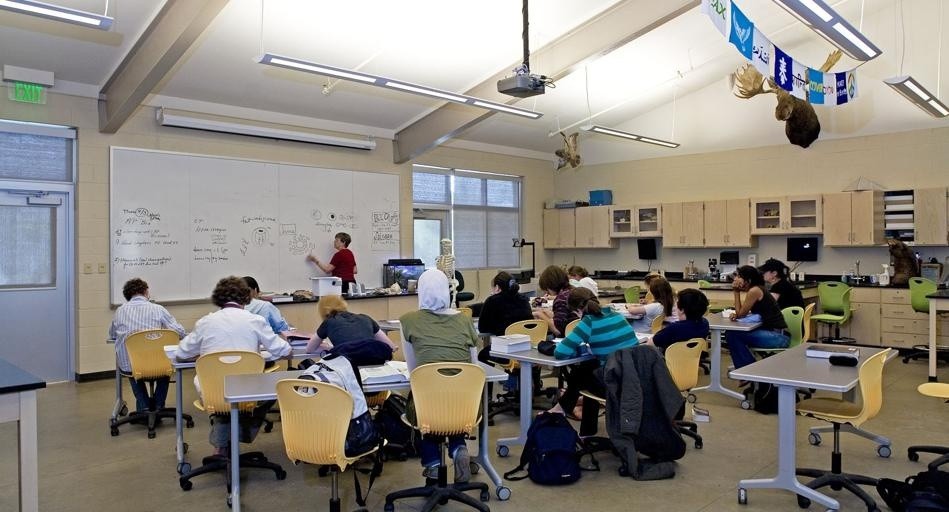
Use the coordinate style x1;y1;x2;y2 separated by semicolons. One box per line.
637;239;657;259
786;237;818;262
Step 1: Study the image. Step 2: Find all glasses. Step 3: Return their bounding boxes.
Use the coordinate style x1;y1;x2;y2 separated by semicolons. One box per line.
577;60;685;154
0;1;115;33
769;1;887;65
880;1;949;121
254;2;547;124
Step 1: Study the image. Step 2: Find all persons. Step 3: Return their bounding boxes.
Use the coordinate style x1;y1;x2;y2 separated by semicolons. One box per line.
241;276;290;410
110;278;189;426
479;271;541;414
174;275;293;456
627;276;679;334
537;287;640;439
757;260;805;358
307;232;359;296
569;266;598;298
398;269;478;482
639;271;661;304
729;265;792;388
639;288;710;357
307;293;398;352
532;265;579;339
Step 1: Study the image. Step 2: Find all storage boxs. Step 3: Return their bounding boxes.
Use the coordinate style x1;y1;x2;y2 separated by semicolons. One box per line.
883;214;915;231
586;188;612;206
884;194;914;210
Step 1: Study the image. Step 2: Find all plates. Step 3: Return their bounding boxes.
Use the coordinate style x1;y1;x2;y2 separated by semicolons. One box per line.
576;205;621;250
608;201;661;239
702;197;759;249
882;184;949;248
658;199;705;250
821;190;886;248
749;194;824;236
542;207;576;250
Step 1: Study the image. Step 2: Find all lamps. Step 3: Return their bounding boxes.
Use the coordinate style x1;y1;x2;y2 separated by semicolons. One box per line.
254;2;547;124
577;60;685;154
769;1;887;65
0;1;115;33
880;1;949;121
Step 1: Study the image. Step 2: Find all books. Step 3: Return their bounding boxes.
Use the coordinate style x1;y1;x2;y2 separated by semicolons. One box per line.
883;214;915;231
586;188;612;206
805;345;860;359
884;194;914;210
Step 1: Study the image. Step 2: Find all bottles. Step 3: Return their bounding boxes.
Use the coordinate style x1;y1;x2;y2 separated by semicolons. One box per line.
758;257;788;275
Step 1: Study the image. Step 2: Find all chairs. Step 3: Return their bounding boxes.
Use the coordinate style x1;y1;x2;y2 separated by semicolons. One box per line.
104;269;820;511
901;277;949;368
908;383;949;471
425;471;444;495
796;348;890;509
453;447;471;486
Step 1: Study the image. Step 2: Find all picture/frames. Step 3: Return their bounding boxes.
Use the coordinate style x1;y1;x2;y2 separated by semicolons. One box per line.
0;355;51;511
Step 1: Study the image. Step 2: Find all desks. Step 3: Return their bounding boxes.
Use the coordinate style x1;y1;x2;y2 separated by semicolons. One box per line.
0;355;51;511
728;342;900;509
925;290;948;382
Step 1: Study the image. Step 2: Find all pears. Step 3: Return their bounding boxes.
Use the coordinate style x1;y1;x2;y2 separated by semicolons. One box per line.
879;264;890;286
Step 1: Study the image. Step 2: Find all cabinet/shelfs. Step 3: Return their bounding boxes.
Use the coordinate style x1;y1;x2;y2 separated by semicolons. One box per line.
542;207;576;250
882;184;949;248
702;197;759;249
658;199;705;250
821;190;886;248
576;205;621;250
608;201;661;239
749;194;824;236
588;273;947;348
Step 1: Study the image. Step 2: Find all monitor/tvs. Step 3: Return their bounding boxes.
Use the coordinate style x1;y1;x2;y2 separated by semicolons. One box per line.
805;345;860;359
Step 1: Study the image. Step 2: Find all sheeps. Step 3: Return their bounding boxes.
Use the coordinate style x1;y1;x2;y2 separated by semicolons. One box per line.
688;259;694;280
856;258;860;276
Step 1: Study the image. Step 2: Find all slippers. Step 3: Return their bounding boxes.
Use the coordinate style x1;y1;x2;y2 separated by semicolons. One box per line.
369;393;420;461
752;347;802;415
320;338;394;367
502;409;600;485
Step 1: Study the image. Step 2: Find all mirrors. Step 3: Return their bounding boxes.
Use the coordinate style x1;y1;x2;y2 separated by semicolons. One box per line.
555;132;584;170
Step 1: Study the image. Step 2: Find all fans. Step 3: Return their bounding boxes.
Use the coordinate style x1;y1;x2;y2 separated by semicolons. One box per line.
109;145;402;309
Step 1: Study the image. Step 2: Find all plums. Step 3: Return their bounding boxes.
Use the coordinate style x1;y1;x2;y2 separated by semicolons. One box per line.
496;74;545;98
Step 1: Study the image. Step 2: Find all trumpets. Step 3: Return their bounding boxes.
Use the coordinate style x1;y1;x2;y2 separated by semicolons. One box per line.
734;49;843;149
